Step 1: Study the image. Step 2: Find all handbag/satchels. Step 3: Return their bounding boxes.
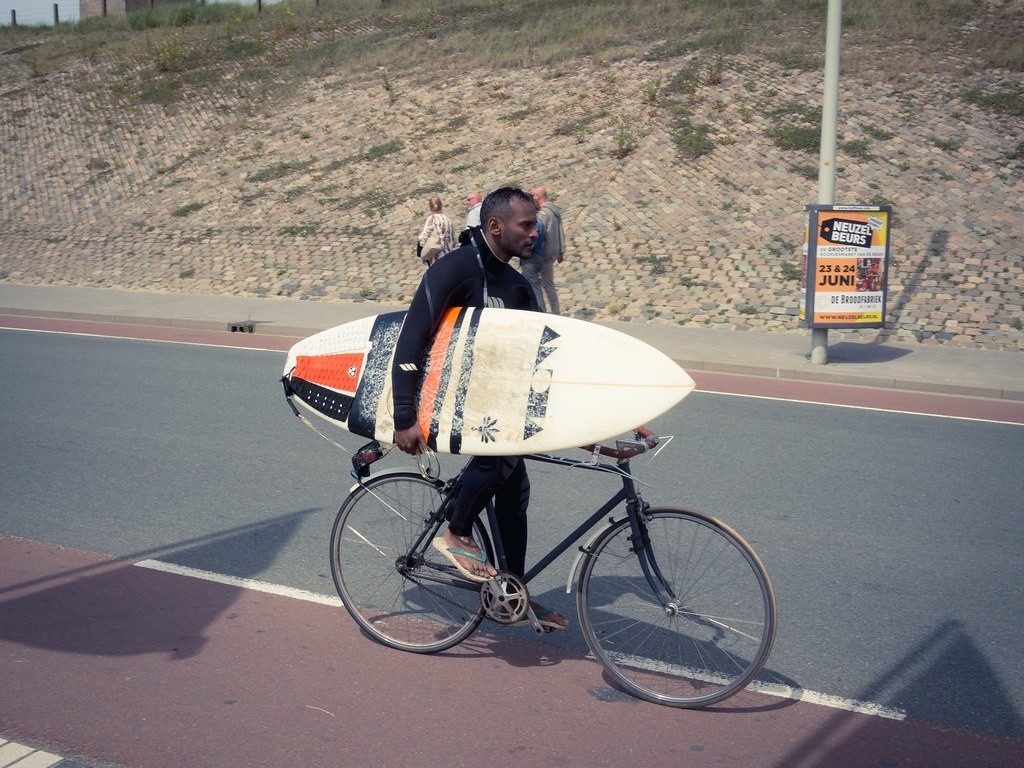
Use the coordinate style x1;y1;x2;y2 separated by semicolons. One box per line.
417;241;425;257
420;215;441;260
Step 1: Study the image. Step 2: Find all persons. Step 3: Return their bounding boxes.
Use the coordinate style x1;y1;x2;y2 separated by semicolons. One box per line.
391;187;659;629
519;192;547;313
465;192;483;231
417;195;456;268
532;187;566;316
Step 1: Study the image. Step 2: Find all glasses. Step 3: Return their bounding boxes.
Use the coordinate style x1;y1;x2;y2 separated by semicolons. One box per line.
467;198;476;201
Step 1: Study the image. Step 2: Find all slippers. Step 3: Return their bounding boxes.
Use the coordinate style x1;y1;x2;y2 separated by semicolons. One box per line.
499;608;565;630
433;538;494;583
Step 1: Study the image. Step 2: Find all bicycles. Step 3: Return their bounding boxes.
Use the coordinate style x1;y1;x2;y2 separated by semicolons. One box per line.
329;422;778;709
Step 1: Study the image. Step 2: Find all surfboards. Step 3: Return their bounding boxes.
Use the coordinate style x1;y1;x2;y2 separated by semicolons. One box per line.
282;306;697;458
